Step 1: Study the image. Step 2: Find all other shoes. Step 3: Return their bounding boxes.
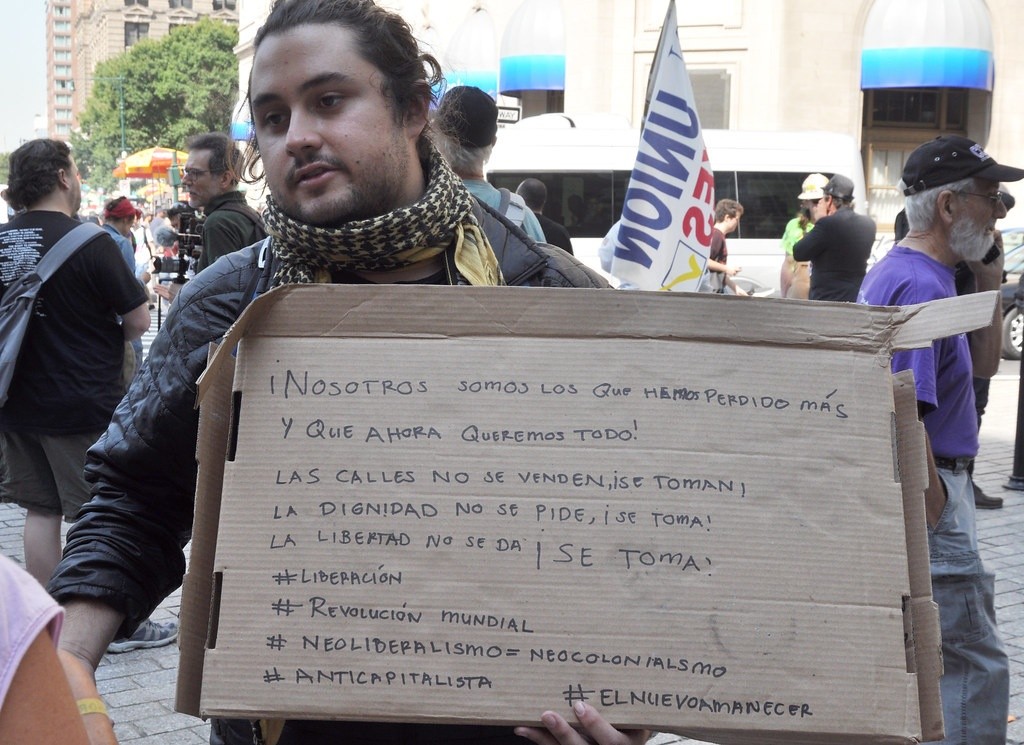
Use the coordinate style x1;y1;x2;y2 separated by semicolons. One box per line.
973;478;1003;509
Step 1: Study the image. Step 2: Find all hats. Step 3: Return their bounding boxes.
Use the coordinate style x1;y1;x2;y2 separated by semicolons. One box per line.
902;134;1024;196
438;86;498;148
157;207;166;214
821;174;854;201
797;173;829;200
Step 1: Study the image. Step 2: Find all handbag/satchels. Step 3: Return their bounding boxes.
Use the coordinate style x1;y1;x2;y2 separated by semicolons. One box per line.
0;223;109;417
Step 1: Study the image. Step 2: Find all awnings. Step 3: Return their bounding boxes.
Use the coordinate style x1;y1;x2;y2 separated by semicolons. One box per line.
231;99;251;140
860;0;992;92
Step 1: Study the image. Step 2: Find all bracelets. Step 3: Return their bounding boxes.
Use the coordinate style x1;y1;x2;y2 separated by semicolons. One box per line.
77;698;107;715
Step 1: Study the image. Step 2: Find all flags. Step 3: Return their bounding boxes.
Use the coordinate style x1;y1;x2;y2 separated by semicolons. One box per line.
610;3;715;290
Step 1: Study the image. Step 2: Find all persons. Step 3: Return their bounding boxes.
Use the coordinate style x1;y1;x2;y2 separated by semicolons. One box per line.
707;199;748;295
436;86;573;256
1;139;177;651
80;197;195;389
780;174;877;303
185;133;267;274
0;554;90;745
857;135;1024;745
48;0;655;745
895;184;1009;509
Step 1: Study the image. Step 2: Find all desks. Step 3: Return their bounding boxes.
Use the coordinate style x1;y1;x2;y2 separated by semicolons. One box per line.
153;284;184;332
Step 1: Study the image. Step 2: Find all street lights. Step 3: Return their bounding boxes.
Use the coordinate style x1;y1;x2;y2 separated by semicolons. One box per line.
66;74;126;153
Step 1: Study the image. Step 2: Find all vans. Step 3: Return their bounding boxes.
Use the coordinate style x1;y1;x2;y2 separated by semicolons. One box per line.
479;111;871;297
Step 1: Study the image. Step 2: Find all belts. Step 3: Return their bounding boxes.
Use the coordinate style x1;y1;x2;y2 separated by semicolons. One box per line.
932;455;976;471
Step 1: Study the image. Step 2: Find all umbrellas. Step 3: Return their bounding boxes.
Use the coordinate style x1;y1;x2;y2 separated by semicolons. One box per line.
112;146;188;210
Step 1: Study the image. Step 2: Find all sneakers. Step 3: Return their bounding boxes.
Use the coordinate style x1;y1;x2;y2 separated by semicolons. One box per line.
106;617;180;653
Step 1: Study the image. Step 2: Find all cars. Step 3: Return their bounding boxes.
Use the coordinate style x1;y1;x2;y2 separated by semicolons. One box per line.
997;227;1023;361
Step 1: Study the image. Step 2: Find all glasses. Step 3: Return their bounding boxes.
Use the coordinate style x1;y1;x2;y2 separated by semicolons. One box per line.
181;168;225;182
948;189;1003;206
